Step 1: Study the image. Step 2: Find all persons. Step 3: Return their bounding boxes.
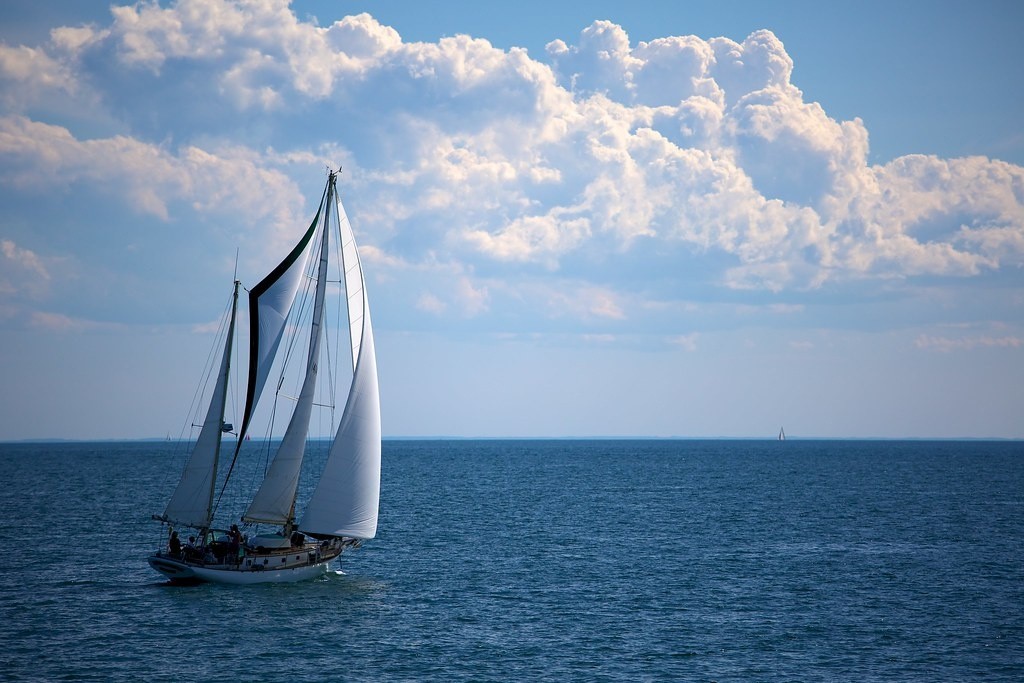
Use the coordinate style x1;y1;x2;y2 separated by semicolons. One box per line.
169;532;182;557
188;537;194;549
230;524;241;564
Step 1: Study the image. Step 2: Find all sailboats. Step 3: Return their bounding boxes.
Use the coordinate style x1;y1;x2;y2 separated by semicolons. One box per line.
244;432;250;442
165;434;171;443
777;426;785;440
146;164;385;587
